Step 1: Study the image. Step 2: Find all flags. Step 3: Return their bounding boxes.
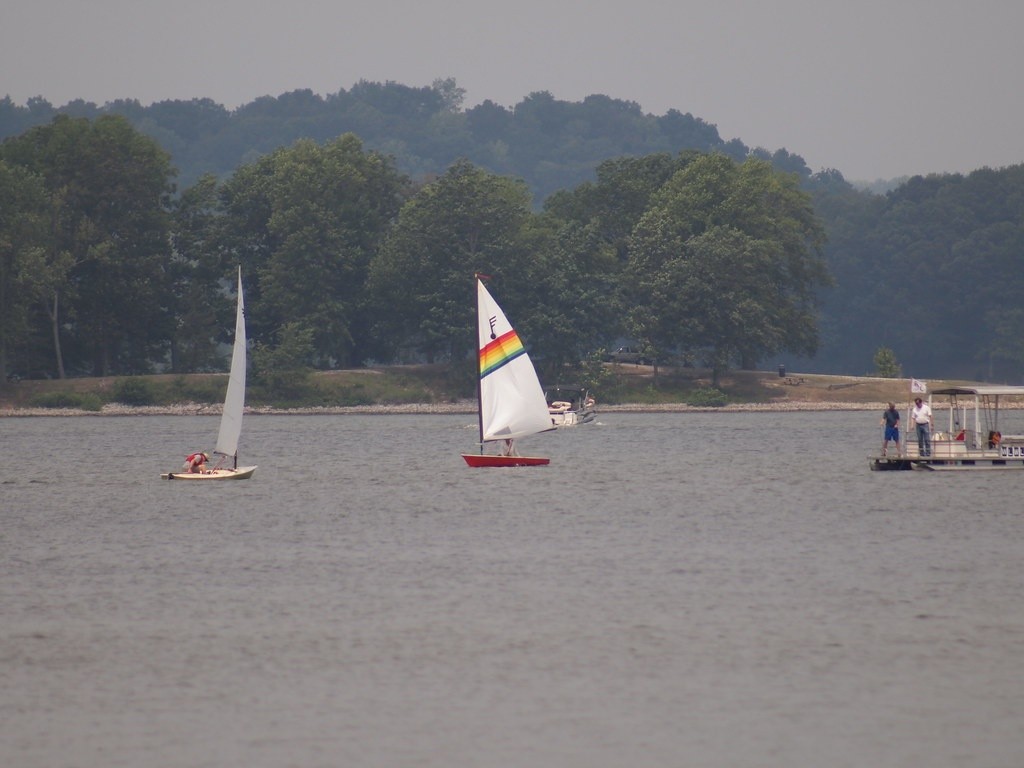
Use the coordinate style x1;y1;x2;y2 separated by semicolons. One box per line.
911;378;927;394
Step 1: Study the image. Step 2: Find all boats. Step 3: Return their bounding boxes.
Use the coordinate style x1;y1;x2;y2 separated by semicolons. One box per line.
537;384;598;433
866;378;1024;471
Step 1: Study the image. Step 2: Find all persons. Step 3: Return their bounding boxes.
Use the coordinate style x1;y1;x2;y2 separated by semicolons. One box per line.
183;452;209;473
880;401;902;458
910;397;935;456
504;438;521;456
568;399;578;413
583;394;595;410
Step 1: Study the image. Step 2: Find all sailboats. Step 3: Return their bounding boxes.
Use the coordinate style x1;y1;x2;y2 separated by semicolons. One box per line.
460;274;557;467
158;263;259;480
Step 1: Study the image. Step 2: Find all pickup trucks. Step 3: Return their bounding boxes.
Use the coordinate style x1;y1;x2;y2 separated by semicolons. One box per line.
610;346;650;365
